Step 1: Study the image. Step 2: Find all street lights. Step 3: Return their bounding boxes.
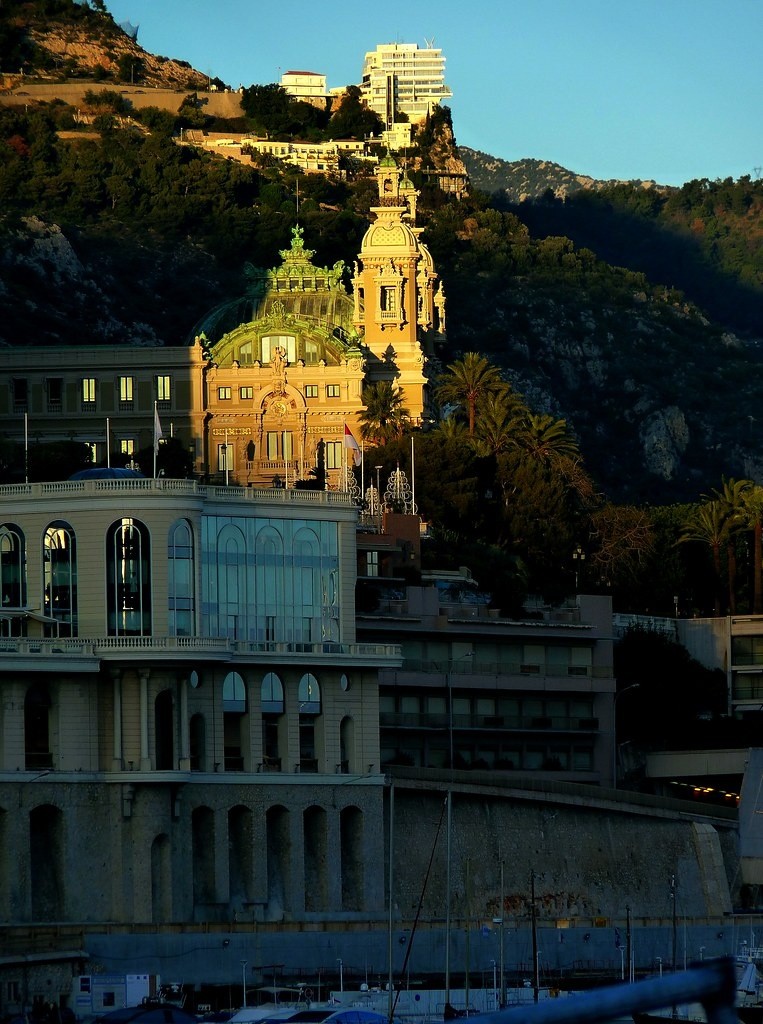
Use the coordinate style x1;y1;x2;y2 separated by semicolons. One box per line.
619;945;628;979
537;950;542;989
657;956;663;977
374;465;383;536
490;959;498;1011
699;946;707;961
573;544;586;594
239;960;248;1010
335;958;344;992
613;683;640;789
448;653;478;784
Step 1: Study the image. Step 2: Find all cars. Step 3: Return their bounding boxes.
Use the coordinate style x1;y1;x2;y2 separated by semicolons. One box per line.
284;1009;398;1024
90;1001;203;1024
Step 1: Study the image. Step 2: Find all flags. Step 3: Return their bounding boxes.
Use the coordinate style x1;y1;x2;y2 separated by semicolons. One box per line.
346;424;361;466
